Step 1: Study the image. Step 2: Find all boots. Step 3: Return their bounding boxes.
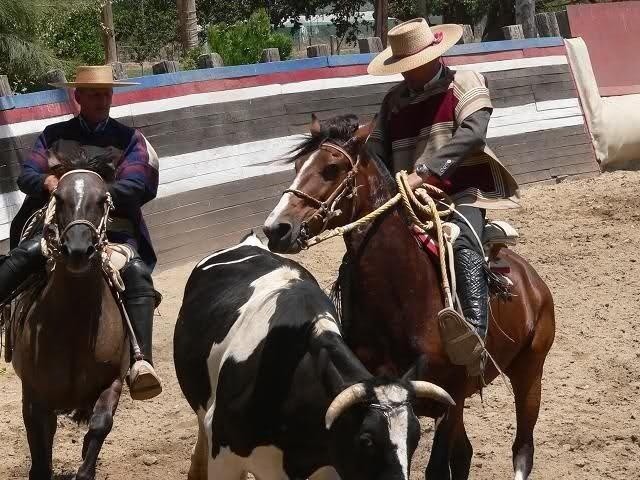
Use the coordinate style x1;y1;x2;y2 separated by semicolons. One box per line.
438;246;492;377
122;260;163;401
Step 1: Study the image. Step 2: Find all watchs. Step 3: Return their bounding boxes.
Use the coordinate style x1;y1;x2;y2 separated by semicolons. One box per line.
416;163;432;179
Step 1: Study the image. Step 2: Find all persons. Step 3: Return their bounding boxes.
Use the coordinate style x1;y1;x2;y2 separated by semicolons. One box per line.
367;19;520;377
0;65;163;399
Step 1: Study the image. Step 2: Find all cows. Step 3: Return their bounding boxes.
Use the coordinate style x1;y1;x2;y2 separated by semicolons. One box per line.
172;227;457;480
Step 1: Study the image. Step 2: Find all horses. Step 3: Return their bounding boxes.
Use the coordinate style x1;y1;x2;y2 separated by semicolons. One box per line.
9;145;131;480
262;111;556;478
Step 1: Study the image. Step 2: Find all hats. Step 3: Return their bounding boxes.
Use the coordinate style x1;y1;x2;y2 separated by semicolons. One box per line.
47;64;142;88
365;16;465;78
